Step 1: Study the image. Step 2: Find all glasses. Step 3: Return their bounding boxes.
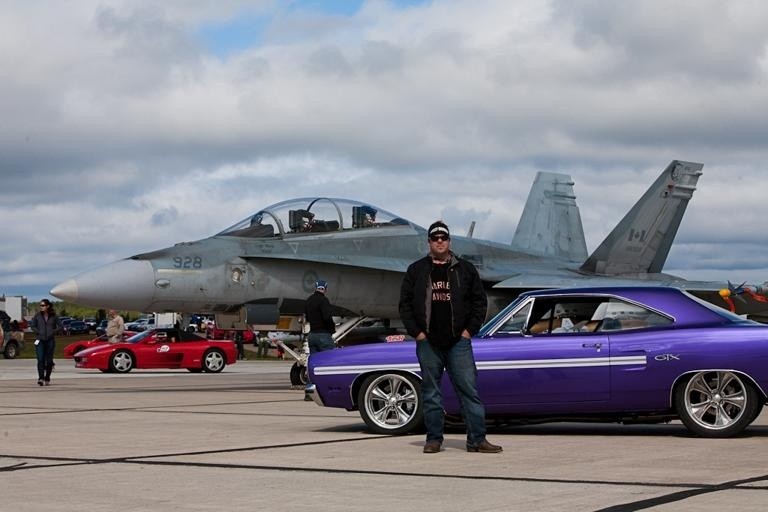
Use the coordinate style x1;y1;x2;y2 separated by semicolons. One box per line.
40;304;49;308
428;236;451;241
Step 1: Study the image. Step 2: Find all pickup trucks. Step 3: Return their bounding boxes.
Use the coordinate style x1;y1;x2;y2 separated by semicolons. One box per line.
0;309;24;361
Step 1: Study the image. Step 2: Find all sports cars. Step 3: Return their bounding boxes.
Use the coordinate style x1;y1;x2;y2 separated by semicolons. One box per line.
308;288;766;434
62;329;136;359
73;330;241;375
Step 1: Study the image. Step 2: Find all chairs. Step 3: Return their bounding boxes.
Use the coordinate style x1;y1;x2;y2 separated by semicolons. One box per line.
593;317;621;332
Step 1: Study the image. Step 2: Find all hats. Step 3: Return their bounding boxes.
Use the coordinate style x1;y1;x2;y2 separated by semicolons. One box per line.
428;221;450;235
315;279;328;289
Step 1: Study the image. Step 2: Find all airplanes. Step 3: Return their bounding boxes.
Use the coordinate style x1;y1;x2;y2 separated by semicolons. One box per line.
48;159;733;328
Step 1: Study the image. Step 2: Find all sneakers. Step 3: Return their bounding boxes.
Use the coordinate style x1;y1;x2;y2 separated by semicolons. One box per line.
263;353;268;358
240;355;246;359
256;353;262;358
45;381;50;386
36;379;44;386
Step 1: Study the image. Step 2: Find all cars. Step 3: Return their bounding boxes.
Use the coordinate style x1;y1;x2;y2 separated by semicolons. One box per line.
26;315;215;336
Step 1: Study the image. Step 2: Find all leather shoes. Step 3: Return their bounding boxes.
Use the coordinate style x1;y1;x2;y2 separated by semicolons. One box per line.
467;437;505;453
422;436;446;453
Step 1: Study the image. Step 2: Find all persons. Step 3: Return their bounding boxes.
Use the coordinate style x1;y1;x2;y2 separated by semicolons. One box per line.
32;303;54;370
256;331;269;358
175;313;214;340
234;330;248;360
105;309;124;338
398;221;501;453
277;345;284;359
31;299;63;385
304;281;360;401
94;309;107;337
